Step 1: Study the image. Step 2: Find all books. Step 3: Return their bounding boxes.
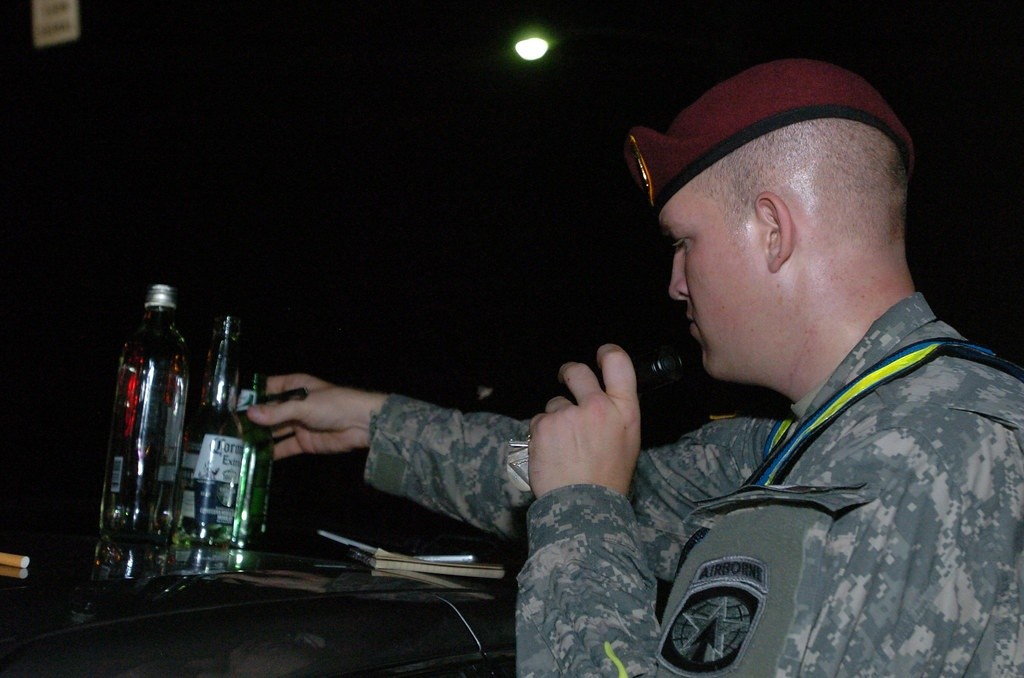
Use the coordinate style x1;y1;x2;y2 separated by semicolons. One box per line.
348;548;505;579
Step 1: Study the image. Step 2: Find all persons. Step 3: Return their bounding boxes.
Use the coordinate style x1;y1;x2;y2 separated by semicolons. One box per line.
247;57;1024;678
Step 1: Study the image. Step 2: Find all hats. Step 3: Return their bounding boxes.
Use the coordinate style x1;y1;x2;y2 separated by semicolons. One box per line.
625;59;913;212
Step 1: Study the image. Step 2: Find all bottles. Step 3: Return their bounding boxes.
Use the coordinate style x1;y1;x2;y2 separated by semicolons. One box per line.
230;371;274;550
164;315;244;548
94;284;191;547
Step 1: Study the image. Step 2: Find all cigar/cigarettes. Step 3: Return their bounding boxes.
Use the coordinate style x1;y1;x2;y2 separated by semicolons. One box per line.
0;565;29;579
0;552;30;569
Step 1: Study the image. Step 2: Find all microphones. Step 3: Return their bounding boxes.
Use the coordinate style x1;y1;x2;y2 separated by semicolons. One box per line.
565;349;684;406
